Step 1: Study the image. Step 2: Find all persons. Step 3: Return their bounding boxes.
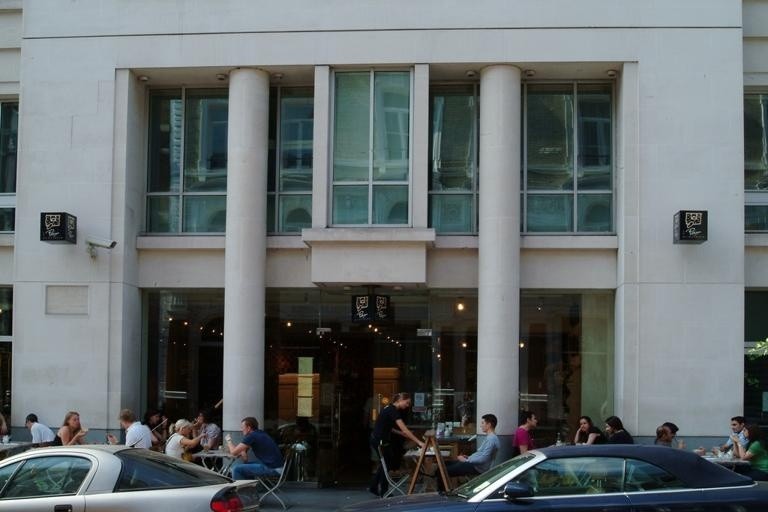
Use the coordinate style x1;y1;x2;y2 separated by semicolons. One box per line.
710;415;749;455
654;422;688;449
192;407;222;451
164;418;207;458
223;416;285;481
511;411;539;458
573;415;607;446
604;415;634;444
287;415;319;481
141;407;168;442
0;413;8;435
24;413;56;444
436;413;501;490
107;408;152;449
729;423;768;481
367;391;426;499
54;410;88;446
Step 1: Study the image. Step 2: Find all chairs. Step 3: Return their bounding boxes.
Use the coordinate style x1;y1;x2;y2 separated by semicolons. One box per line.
256;449;292;510
378;447;409;498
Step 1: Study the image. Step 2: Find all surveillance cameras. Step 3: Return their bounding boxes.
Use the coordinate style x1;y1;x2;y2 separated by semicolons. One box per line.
85;235;117;249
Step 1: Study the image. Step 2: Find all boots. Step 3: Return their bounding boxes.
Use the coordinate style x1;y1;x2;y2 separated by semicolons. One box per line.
369;473;380;496
380;480;393;497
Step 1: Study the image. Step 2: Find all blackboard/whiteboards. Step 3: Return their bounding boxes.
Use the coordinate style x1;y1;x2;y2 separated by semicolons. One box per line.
430;432;452;491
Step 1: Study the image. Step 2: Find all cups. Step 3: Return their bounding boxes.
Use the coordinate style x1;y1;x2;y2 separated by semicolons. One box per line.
219;446;225;454
728;450;734;460
3;436;8;444
204;444;209;453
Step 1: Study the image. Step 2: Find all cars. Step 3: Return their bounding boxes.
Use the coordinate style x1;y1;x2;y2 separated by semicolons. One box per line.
336;444;767;512
0;442;260;512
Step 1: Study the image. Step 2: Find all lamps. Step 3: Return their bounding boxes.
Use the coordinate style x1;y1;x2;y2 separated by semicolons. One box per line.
351;284;391;324
39;212;78;245
674;210;709;245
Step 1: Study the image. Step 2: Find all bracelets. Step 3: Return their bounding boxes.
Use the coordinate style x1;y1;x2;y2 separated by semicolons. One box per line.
737;444;744;448
162;427;166;430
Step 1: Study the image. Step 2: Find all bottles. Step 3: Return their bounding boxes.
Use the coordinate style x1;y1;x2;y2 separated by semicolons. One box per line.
674;437;686;450
556;432;561;445
444;425;450;438
436;424;442;439
